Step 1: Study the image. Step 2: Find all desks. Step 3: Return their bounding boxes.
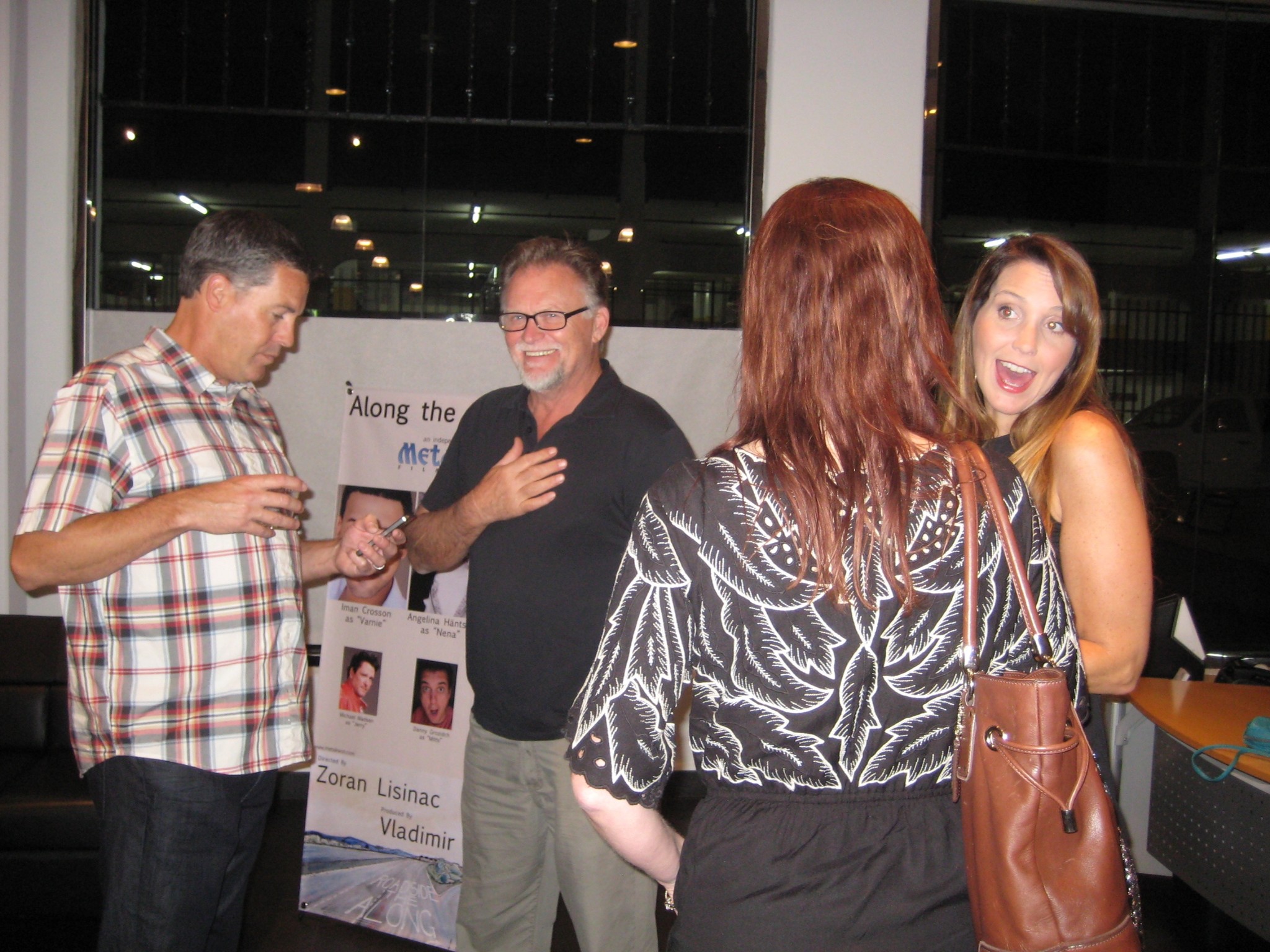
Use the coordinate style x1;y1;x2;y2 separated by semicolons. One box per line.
1128;678;1270;940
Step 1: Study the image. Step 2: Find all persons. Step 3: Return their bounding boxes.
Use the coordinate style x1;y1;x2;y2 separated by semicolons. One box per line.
944;230;1151;693
408;551;469;619
10;208;407;952
411;658;454;731
339;651;380;713
559;178;1087;952
325;485;414;612
409;235;700;952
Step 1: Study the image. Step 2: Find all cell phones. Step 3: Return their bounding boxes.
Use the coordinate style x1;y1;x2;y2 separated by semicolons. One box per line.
355;512;413;557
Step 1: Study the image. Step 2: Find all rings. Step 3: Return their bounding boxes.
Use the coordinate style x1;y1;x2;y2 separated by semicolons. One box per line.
373;564;384;570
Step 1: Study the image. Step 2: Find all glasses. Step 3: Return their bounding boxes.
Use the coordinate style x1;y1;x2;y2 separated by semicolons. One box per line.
499;302;593;331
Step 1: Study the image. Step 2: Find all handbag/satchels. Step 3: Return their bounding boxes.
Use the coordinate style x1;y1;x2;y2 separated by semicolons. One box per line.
948;441;1143;952
